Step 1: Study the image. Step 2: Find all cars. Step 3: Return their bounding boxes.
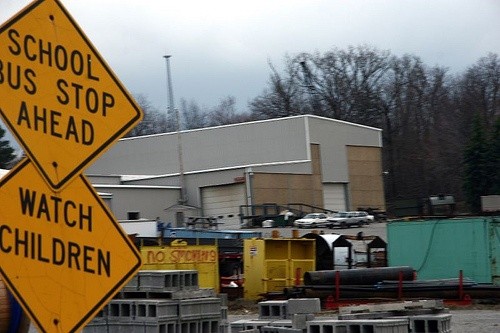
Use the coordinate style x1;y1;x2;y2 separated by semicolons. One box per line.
293;213;330;228
325;211;363;229
357;212;375;225
